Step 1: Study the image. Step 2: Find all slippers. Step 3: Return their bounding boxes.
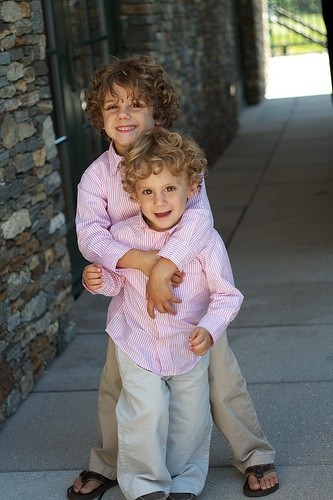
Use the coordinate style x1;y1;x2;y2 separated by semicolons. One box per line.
66;470;118;500
243;464;279;498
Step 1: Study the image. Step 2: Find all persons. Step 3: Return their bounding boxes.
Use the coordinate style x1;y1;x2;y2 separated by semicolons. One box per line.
81;126;244;500
66;55;280;500
